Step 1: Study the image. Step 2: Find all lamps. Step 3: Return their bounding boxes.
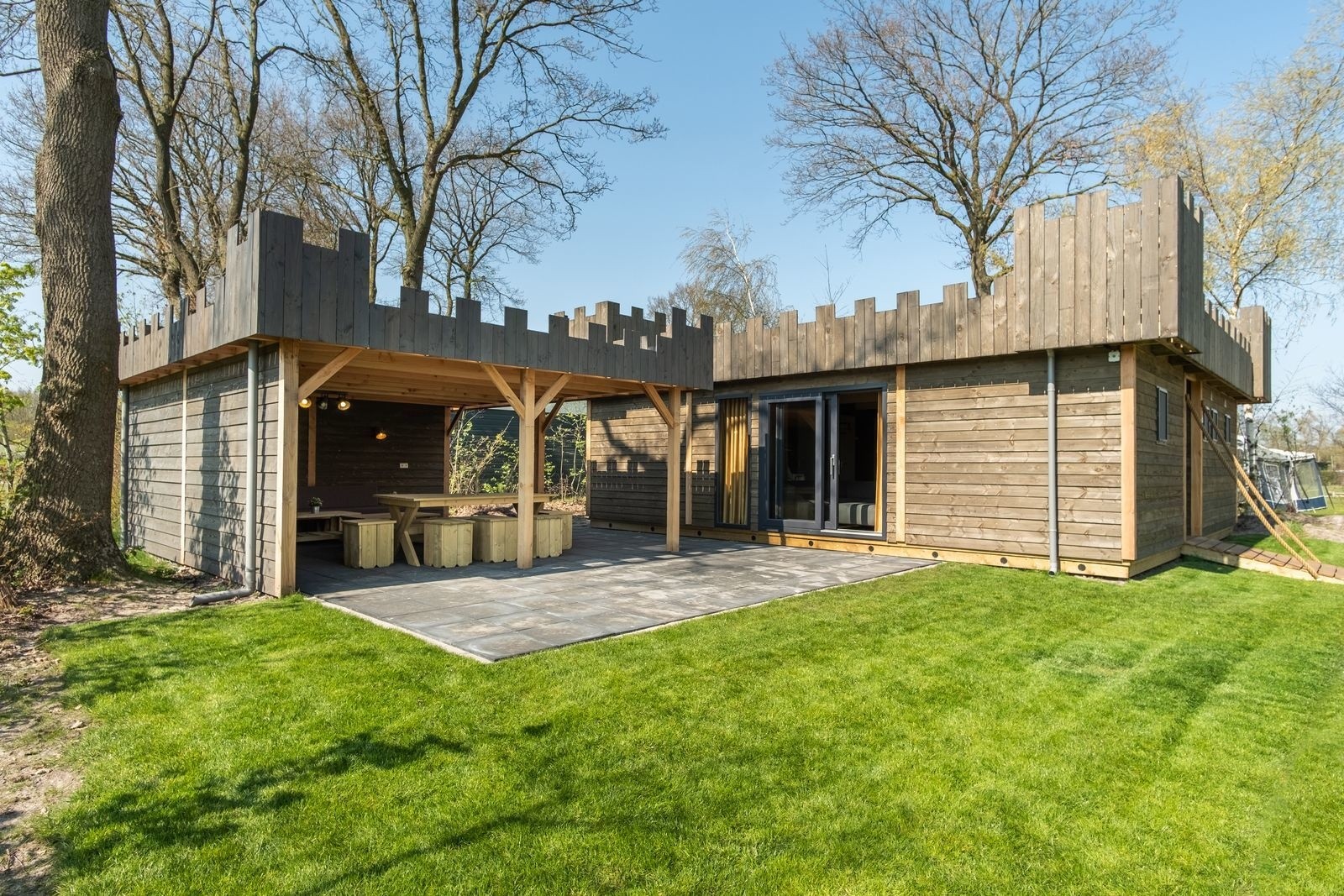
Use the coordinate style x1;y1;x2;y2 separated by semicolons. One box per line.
316;392;329;409
376;430;387;439
299;396;312;408
336;392;351;410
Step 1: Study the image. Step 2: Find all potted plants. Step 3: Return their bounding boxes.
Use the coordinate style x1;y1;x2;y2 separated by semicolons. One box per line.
309;497;322;514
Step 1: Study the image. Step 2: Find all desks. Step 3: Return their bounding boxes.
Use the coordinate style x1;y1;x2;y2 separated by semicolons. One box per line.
296;510;365;542
375;493;559;567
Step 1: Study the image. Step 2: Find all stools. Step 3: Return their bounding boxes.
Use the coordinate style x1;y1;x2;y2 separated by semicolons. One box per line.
342;510;574;568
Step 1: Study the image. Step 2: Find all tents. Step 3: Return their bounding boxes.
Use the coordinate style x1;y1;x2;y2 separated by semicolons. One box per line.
1236;435;1335;514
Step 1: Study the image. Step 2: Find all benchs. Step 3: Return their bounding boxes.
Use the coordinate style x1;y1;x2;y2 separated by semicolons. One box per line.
311;486;390;513
